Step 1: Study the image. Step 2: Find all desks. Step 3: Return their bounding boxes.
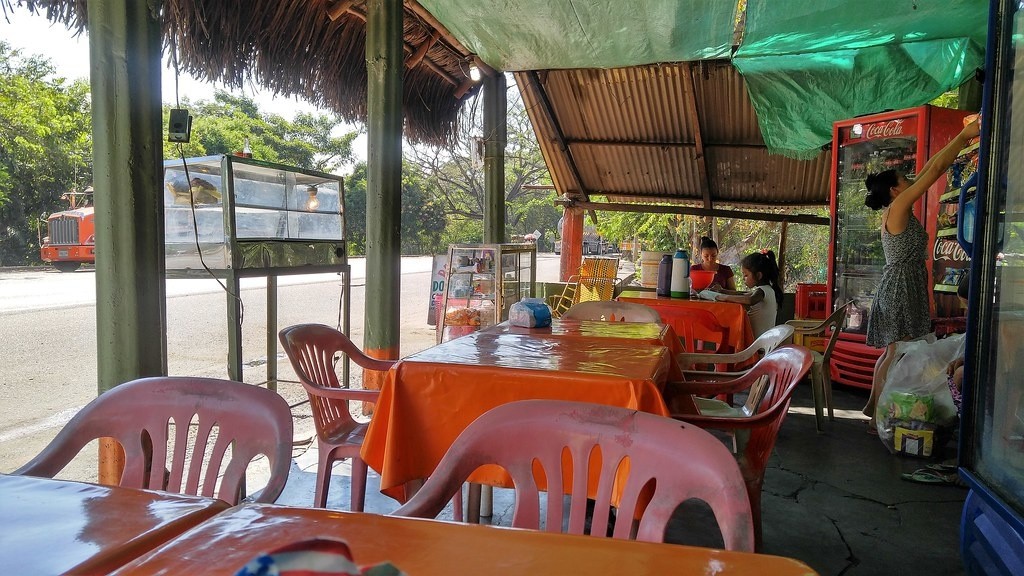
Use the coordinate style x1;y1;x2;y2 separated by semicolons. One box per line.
0;474;235;576
359;319;676;518
106;503;818;575
616;290;758;408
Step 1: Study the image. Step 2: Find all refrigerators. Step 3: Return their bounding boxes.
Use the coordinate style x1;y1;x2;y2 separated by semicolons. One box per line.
824;103;977;399
955;0;1024;576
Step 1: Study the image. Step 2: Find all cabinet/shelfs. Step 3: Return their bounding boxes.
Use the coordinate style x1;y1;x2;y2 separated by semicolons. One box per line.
933;135;981;297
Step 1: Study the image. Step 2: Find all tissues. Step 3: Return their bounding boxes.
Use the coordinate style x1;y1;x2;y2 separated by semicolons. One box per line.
508;296;553;328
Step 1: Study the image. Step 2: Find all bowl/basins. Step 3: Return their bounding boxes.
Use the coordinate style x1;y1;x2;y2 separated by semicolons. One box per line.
690;270;717;294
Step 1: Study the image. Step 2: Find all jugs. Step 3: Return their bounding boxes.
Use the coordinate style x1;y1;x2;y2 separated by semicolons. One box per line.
670;248;689;298
656;255;672;297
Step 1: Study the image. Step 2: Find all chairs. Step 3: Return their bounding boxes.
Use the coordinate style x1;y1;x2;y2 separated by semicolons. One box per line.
666;345;814;549
676;324;794;455
10;376;293;505
553;258;620;320
784;301;853;436
558;300;661;322
279;323;398;512
385;399;755;553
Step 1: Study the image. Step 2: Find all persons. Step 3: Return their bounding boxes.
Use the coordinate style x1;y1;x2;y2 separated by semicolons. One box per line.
714;249;784;354
689;237;735;295
864;118;980;435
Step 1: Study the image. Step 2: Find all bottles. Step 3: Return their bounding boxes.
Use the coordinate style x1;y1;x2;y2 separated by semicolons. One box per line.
840;234;887;266
848;205;883;227
997;222;1024;460
848;142;915;179
831;284;878;333
